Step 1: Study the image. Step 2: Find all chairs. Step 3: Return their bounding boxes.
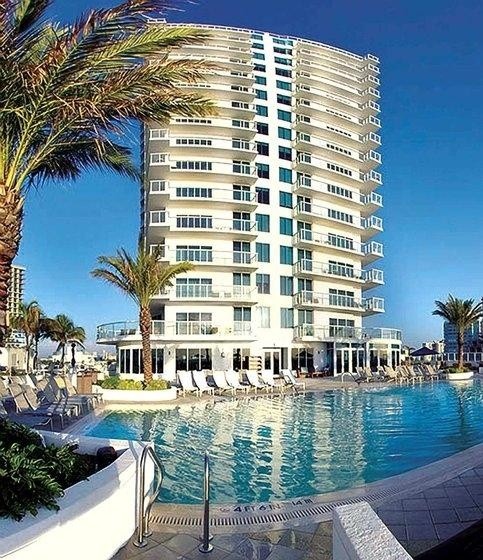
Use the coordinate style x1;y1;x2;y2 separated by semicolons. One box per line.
0;371;104;430
172;367;306;399
354;363;446;386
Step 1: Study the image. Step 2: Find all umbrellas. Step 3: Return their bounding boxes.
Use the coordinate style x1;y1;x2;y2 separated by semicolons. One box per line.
410;346;440;364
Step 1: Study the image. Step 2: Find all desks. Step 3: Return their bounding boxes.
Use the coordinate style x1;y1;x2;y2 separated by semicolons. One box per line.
300;370;324;379
